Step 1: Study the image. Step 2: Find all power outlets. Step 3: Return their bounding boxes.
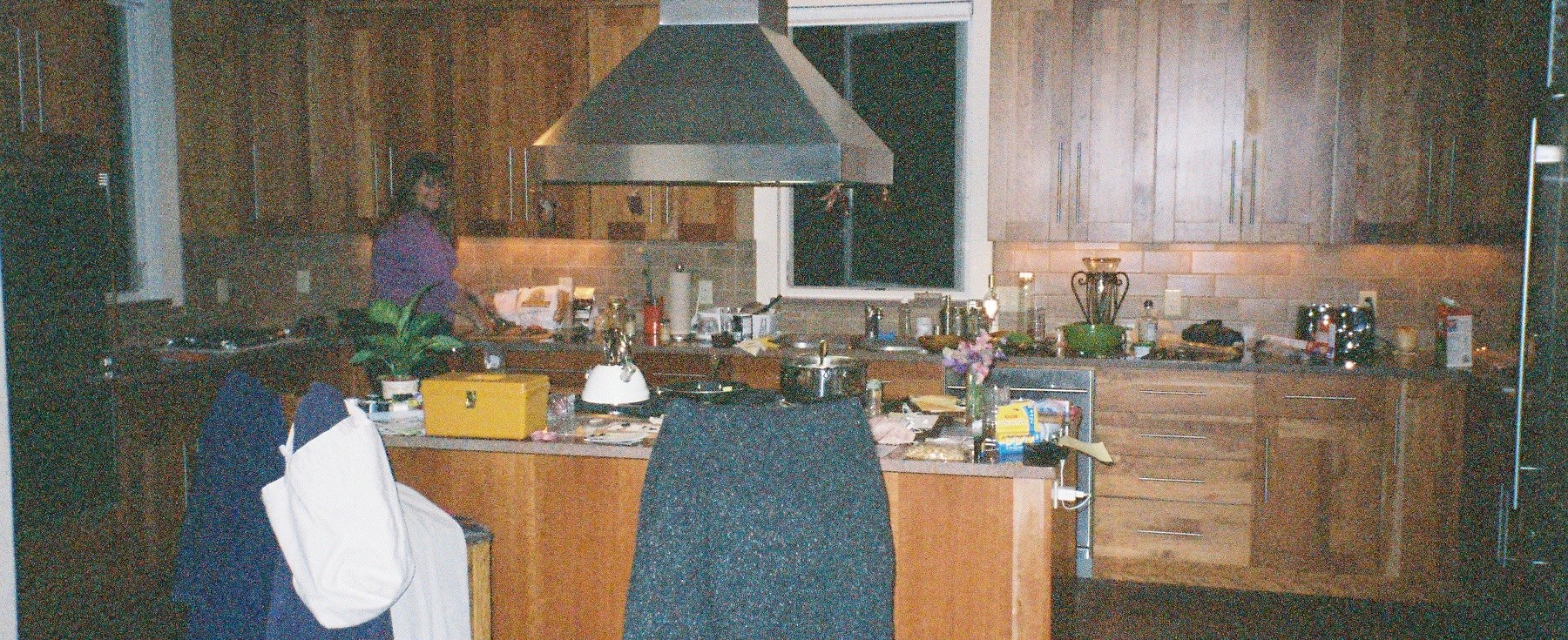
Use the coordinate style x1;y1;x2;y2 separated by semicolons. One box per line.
698;279;713;304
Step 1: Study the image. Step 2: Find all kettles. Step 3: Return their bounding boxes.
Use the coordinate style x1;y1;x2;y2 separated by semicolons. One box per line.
582;329;649;406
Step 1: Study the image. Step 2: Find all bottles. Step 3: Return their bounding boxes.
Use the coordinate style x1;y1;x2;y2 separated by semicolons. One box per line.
984;274;999;333
898;299;912;340
1138;300;1158;343
866;379;883;417
604;296;630;334
643;296;662;335
982;366;1011;438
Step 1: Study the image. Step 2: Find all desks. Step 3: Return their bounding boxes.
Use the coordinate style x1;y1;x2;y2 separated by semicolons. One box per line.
366;394;1088;640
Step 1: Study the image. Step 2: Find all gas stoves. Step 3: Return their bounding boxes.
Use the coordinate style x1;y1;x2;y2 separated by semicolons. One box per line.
569;381;907;424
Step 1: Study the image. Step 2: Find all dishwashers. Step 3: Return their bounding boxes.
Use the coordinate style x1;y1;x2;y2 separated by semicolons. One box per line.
945;366;1092;578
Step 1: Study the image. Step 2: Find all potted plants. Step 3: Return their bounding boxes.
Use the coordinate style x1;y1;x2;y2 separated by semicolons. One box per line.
346;279;468;398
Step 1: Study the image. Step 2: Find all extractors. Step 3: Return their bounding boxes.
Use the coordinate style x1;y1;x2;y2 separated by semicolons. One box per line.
530;0;895;190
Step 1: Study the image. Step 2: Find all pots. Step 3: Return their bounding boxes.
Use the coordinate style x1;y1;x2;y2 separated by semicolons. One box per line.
777;354;869;402
660;379;750;402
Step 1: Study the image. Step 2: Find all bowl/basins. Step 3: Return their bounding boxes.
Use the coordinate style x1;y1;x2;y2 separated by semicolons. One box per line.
1062;320;1128;360
1133;340;1155;358
1308;305;1371;349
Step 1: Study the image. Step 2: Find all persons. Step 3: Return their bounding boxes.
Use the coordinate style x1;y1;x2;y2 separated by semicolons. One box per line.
371;152;491;335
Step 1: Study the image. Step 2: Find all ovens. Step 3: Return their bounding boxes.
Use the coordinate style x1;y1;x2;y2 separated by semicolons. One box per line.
2;151;113;534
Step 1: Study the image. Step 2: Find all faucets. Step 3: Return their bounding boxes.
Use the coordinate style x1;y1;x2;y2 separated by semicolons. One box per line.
864;304;883;339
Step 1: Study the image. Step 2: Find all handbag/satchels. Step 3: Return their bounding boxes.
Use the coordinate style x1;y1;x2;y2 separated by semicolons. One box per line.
262;397;418;631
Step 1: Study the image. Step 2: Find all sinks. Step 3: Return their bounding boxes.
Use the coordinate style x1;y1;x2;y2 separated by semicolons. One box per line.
851;333;928;352
773;332;852;349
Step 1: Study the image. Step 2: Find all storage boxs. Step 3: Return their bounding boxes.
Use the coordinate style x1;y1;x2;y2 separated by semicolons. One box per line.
422;369;553;443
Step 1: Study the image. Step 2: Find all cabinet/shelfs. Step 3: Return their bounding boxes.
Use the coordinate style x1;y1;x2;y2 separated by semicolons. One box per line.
984;0;1541;255
1093;370;1475;600
80;347;950;622
173;0;740;256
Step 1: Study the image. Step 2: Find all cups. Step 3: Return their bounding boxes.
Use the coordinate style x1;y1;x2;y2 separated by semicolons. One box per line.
546;392;576;440
